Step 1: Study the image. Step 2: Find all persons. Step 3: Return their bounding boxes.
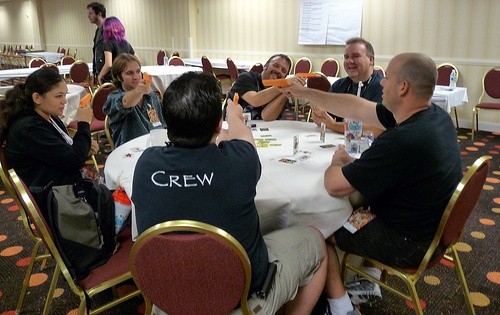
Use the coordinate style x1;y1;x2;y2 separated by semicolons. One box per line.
0;67;94;232
97;16;140;86
222;53;307;122
131;71;328;314
277;51;463;315
102;52;167;149
86;2;106;84
310;37;384;140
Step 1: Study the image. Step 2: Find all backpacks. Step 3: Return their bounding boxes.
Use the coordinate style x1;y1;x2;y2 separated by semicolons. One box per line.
28;178;131;280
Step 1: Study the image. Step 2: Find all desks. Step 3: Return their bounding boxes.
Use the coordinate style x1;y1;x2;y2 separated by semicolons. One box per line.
183;58;255;76
0;63;93;82
0;85;88;127
105;118;371;241
288;75;468;111
140;65;203;92
26;52;64;64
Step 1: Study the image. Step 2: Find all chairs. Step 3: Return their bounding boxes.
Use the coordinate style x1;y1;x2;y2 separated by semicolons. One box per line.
0;150;60;314
127;219;257;315
0;44;114;169
156;50;264;84
436;63;459;86
341;154;492;315
288;57;340;122
8;168;139;315
472;66;500;138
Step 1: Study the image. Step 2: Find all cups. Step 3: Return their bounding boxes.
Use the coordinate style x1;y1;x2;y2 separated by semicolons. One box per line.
360;131;375;153
150;129;166;147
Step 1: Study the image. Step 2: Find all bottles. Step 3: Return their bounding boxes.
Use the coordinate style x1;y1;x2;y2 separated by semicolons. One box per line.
112;187;132;235
449;70;456;91
344;117;362;157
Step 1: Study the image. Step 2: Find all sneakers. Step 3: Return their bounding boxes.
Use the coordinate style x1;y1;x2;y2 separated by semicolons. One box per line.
323;301;362;315
344;274;383;310
